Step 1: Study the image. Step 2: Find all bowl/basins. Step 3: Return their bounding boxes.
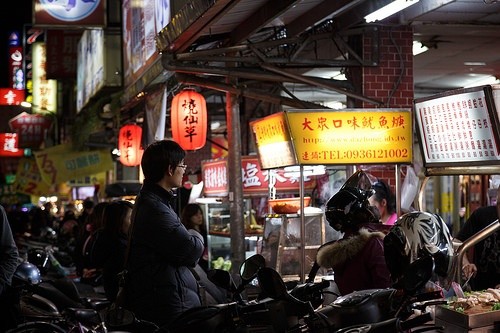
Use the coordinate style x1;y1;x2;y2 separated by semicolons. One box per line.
268;196;311;214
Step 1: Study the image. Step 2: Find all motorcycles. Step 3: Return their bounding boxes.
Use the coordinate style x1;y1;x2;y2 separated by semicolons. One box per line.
8;224;448;333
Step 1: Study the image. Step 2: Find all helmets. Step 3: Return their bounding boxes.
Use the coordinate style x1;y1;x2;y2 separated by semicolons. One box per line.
14;261;40;284
325;186;379;235
384;210;462;278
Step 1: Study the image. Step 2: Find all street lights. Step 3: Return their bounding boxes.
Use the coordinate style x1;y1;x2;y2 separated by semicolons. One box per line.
21;102;59;147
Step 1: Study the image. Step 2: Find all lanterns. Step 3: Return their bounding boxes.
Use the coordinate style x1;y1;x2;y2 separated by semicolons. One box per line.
171;88;207;154
118;122;143;167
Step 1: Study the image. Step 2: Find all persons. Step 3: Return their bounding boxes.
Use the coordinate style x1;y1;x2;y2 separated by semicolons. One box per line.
316;170;391;296
0;140;209;333
368;179;397;225
452;185;500;292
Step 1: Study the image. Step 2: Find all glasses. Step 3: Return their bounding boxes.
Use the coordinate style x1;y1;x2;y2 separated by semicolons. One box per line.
177;165;187;171
